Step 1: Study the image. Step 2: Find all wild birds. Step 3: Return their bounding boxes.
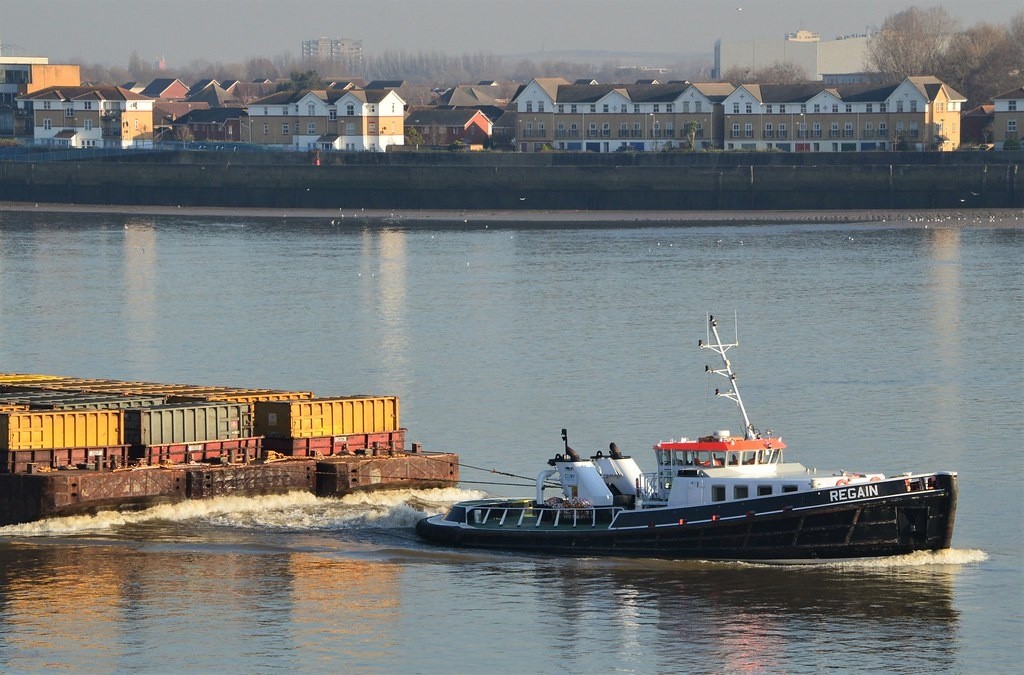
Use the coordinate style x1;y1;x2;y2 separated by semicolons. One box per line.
1;0;1024;277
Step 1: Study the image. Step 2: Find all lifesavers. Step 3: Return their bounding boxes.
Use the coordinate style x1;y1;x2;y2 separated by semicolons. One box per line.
868;476;885;483
833;477;852;489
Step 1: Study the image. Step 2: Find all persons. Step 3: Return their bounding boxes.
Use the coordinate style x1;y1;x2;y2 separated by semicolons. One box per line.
566;447;581;462
747;453;762;465
732;454;738;464
608;443;624;459
703;453;722;467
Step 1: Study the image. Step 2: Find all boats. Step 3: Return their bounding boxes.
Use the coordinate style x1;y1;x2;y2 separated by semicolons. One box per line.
414;307;960;560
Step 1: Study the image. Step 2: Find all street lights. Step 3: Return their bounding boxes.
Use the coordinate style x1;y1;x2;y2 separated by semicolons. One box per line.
250;120;254;143
414;120;419;134
941;118;944;142
212;119;216;142
73;117;78;149
341;120;344;149
649;113;656;150
518;120;522;152
800;113;806;152
